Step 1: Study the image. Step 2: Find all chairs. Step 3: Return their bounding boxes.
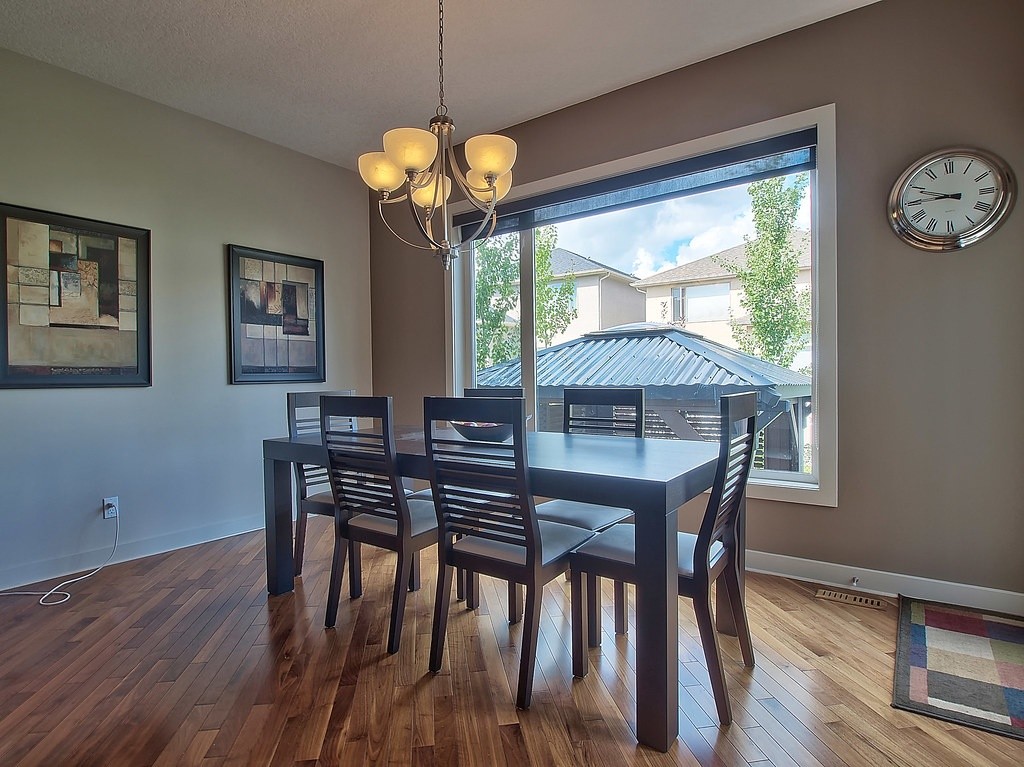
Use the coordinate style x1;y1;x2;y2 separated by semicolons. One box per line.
287;390;421;600
423;397;601;708
406;388;524;601
564;390;758;723
319;393;480;655
515;388;645;646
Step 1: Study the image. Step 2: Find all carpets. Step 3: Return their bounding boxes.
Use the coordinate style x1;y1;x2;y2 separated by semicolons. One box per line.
890;593;1024;739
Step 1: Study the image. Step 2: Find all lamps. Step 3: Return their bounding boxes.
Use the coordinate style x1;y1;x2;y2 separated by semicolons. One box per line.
357;0;518;271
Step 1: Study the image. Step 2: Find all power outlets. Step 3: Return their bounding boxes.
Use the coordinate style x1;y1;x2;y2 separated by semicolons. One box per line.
104;496;119;519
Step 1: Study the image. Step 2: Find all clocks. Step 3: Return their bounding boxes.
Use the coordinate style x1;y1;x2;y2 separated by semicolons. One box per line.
884;144;1017;253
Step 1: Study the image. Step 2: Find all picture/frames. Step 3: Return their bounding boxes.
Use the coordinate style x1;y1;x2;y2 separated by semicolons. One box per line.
226;243;327;384
0;202;152;390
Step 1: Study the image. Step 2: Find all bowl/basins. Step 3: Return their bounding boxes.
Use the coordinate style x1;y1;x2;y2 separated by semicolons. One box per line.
450;413;532;442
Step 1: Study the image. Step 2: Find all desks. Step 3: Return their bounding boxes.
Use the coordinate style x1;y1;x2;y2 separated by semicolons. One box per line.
262;425;720;752
